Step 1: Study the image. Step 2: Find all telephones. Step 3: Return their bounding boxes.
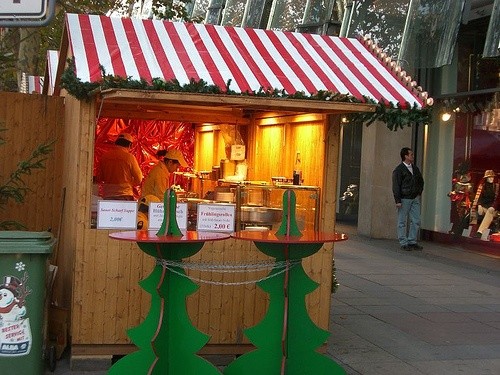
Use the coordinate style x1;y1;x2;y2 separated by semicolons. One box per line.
197;203;235;232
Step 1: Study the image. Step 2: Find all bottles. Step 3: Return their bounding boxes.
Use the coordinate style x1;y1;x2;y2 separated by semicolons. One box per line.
293;153;302;186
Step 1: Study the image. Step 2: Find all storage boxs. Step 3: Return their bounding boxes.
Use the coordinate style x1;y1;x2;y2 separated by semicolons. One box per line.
225;144;245;160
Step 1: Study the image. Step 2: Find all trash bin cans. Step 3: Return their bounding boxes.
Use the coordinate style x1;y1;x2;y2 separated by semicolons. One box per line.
0;230;57;375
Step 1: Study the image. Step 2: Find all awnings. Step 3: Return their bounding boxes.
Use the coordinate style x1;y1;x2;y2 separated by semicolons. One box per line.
21;12;427;115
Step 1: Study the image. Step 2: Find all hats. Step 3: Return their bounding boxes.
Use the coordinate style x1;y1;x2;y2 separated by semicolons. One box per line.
483;170;496;179
165;149;189;167
117;133;132;142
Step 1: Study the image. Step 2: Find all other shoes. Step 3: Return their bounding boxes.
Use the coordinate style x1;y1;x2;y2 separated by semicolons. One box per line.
473;233;482;238
400;244;424;251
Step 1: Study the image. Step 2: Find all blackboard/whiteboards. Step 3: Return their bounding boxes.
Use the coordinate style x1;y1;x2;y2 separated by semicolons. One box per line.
97;200;139;230
148;201;188;231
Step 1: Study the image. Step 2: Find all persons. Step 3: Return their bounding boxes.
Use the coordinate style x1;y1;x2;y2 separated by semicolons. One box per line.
472;170;500;237
96;132;143;201
147;149;182;201
136;195;161;231
392;147;424;250
